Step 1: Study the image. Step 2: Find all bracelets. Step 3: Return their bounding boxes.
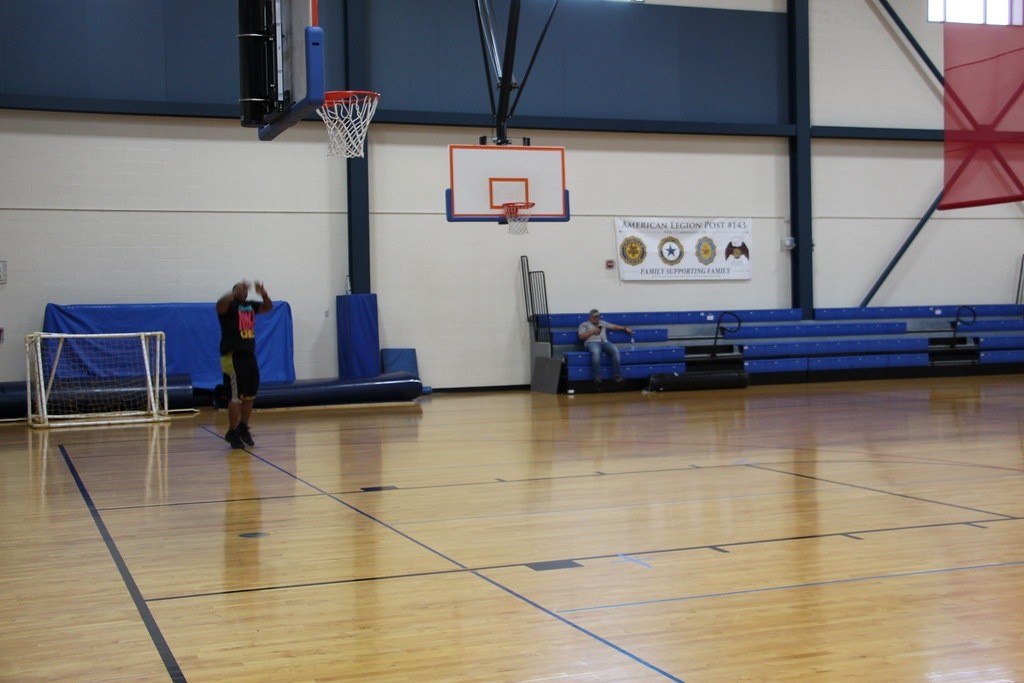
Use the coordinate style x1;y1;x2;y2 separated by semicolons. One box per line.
624;327;626;332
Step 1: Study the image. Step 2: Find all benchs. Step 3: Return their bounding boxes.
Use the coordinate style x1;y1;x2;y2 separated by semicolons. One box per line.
527;303;1024;395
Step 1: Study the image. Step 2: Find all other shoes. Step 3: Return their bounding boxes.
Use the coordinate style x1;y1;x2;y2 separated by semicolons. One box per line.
594;378;601;383
615;377;624;382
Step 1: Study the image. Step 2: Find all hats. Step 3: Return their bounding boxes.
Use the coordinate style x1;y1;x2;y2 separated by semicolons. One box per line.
590;310;600;317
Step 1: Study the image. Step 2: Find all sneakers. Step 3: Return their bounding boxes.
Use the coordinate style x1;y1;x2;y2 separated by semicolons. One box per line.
225;422;255;449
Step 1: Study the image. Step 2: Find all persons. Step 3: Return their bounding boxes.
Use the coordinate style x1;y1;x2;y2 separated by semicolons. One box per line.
578;310;633;389
216;280;273;450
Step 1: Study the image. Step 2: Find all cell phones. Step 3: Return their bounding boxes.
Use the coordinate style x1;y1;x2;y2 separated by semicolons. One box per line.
598;325;602;330
